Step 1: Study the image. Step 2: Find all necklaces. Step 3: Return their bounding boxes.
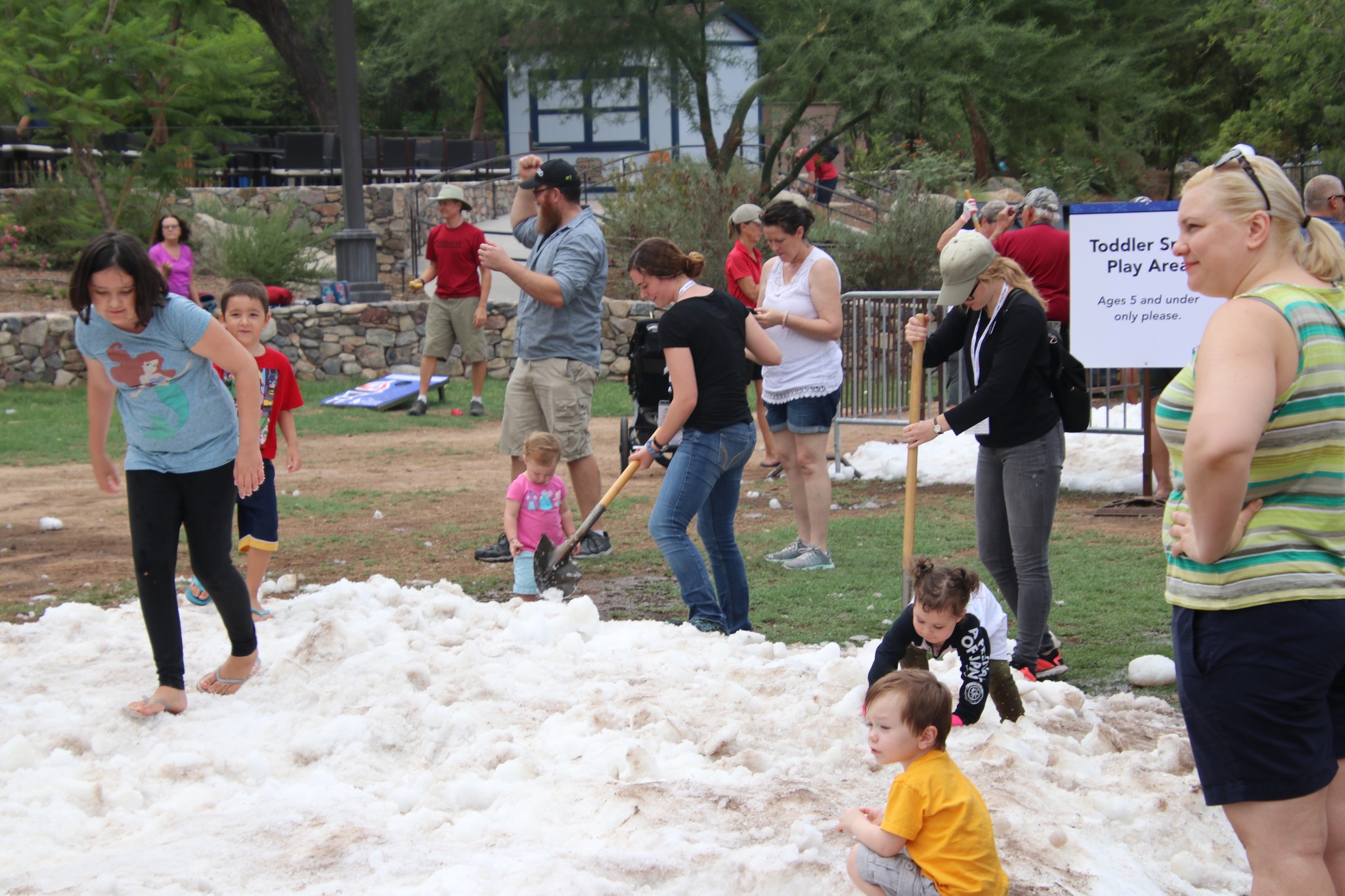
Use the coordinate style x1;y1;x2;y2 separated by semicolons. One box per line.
676;279;696;302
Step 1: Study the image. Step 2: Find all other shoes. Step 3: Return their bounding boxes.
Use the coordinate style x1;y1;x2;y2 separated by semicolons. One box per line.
761;460;781;467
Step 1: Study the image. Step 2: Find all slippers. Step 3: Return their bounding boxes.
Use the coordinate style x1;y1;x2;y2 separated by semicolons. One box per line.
252;607;273;622
122;695;181;719
196;657;261;695
185;576;210;606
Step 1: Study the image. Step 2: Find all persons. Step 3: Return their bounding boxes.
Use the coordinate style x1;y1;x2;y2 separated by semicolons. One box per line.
862;556;1026;728
835;668;1009;896
1299;174;1345;249
184;282;304;622
148;213;202;310
474;153;612;559
627;237;781;637
900;173;1185;683
791;146;839;207
503;430;581;602
69;230;266;721
725;203;781;467
1155;145;1345;896
405;184;491;416
749;199;844;572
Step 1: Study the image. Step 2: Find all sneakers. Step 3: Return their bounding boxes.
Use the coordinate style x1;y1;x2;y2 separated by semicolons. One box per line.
407;401;427;416
469;400;484;415
570;530;614;559
474;533;513;562
782;546;835;572
688;619;724;636
1008;658;1037;682
1034;647;1069;678
764;537;811;562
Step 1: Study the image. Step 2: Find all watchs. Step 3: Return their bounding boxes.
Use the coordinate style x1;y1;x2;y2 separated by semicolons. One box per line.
934;417;944;435
654;436;669;452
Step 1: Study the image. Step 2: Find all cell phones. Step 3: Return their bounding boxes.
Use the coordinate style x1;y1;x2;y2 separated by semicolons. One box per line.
746;306;758;314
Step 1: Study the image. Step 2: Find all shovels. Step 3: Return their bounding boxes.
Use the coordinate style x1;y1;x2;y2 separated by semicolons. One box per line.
889;313;932;671
533;425;652;599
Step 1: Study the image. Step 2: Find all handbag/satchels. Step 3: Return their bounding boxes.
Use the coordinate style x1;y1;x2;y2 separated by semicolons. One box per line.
320;280;351;306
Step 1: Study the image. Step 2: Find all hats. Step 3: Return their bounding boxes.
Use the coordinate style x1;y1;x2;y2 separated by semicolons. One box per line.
1011;186;1059;213
428;184;472;210
936;230;995;305
519;158;581;189
732;204;763;225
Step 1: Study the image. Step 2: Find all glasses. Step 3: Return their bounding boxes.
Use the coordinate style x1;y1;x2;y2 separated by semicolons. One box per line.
162;225;179;230
1213;148;1271;220
534;186;562;197
967;279;981;301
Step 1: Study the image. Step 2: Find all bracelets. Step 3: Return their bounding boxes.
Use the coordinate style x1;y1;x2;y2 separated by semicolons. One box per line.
647;440;664;458
781;312;789;329
417;278;425;287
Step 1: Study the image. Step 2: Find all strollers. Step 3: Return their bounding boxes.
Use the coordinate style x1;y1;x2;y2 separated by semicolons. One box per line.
618;317;683;474
